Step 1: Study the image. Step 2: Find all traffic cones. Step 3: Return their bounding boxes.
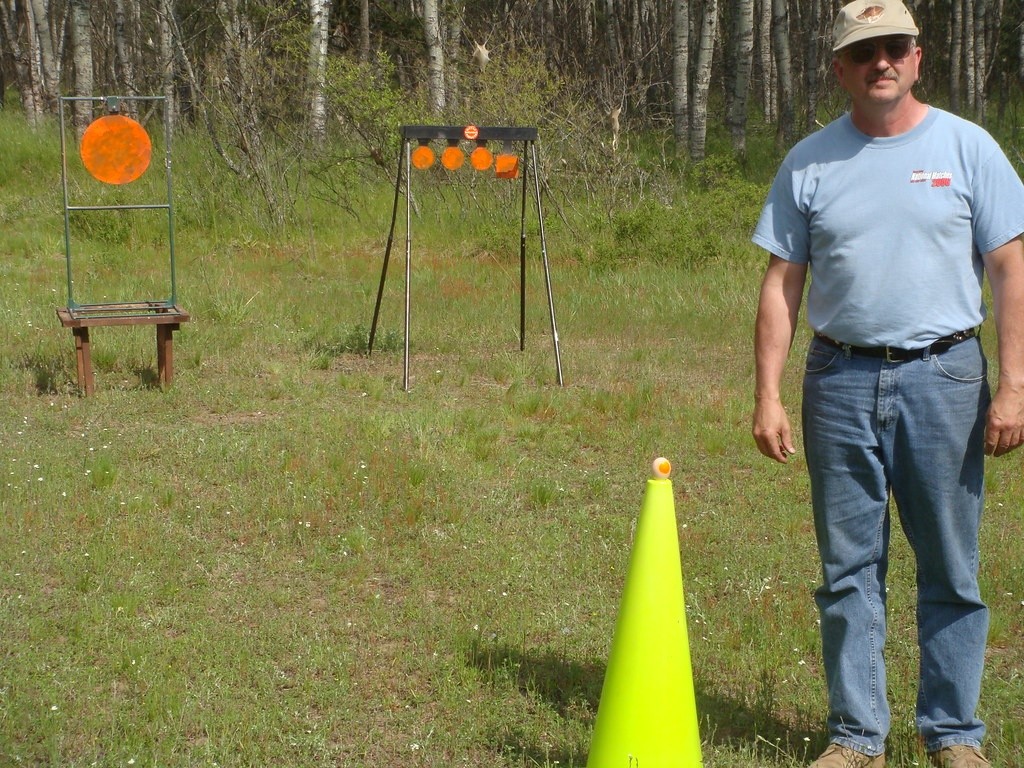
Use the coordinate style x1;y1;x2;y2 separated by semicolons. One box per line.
585;478;704;768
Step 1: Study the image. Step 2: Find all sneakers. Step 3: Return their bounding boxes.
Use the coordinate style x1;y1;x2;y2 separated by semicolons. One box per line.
930;744;992;768
808;744;886;768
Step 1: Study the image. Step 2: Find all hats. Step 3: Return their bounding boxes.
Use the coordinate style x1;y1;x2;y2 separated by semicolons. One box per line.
832;0;919;51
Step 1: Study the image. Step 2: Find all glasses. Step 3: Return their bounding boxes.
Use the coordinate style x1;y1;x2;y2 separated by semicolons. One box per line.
838;39;918;64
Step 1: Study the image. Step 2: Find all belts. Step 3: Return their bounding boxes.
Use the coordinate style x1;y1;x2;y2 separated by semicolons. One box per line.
815;328;976;363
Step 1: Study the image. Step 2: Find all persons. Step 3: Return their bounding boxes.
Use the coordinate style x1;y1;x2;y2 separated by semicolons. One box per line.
750;0;1024;768
